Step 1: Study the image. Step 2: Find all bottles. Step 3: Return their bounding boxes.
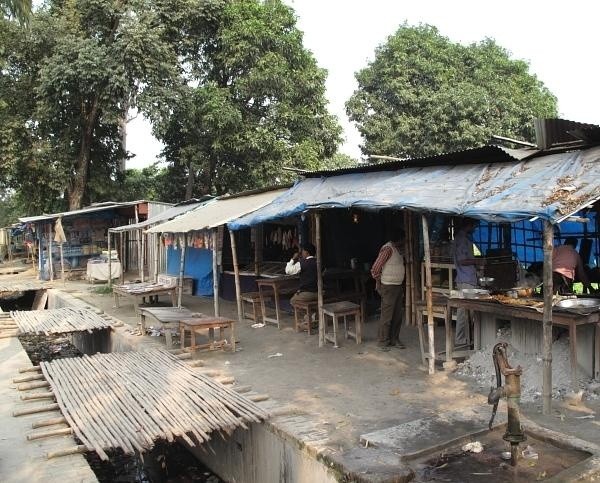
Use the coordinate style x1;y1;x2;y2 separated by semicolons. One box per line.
429;239;454;256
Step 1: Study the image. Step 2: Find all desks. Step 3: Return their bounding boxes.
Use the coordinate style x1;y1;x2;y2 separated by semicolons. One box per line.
255;274;299;328
113;282;177;317
445;294;600;391
324;262;372;295
179;315;235;355
135;304;197;347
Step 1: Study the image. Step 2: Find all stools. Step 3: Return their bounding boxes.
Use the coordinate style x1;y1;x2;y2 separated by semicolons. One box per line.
414;300;471;365
320;301;362;348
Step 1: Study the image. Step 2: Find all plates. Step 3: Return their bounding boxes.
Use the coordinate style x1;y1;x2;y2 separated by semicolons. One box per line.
430;256;453;264
116;282;168;294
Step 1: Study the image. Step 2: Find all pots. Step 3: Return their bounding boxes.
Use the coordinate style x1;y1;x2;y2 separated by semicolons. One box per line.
346;256;360;273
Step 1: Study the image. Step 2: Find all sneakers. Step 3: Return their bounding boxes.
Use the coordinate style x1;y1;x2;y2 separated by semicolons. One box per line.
304;312;318;330
392;340;405;348
376;344;390;351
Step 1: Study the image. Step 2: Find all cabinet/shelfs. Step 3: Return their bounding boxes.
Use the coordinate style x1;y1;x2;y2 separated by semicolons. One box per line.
421;262;459;301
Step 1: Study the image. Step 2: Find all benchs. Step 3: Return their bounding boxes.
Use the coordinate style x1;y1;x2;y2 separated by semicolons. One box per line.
294;289;366;335
241;290;286;322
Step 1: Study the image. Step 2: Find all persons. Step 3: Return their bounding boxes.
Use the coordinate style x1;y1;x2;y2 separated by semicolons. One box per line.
552;236;595;296
284;244;326;329
451;218;480;347
371;228;405;350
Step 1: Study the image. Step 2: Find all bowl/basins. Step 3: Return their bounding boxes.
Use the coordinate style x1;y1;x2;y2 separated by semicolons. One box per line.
557;298;600;313
512;286;530;291
497;288;511;294
479;277;496;287
